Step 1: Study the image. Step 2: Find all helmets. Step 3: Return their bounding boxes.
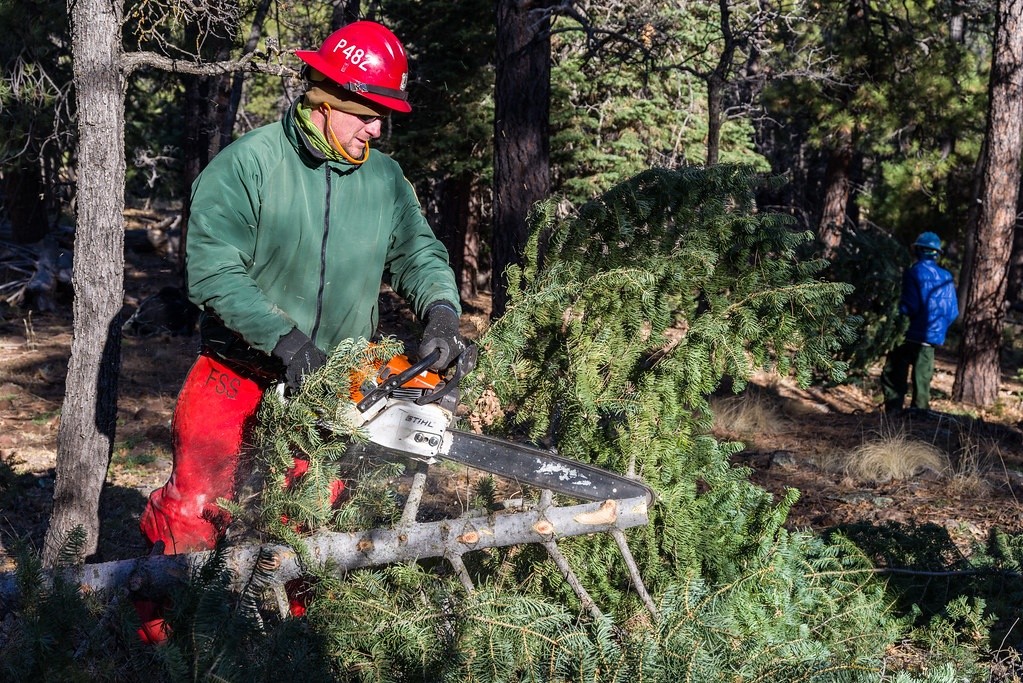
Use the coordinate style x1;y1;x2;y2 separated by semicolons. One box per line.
913;230;946;256
295;20;414;117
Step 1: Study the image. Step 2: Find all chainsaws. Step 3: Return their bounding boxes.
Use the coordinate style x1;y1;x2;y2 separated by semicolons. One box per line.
354;346;658;514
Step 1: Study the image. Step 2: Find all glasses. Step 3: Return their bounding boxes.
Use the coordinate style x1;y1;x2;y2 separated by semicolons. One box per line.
338;103;388;127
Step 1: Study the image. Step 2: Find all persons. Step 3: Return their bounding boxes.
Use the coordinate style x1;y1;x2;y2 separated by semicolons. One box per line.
879;232;958;415
134;18;464;645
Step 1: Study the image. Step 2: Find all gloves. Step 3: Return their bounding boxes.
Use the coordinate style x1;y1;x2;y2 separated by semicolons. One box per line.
267;327;331;391
417;302;465;374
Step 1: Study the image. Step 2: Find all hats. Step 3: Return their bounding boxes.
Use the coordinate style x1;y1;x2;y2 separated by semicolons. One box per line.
303;67;387;116
